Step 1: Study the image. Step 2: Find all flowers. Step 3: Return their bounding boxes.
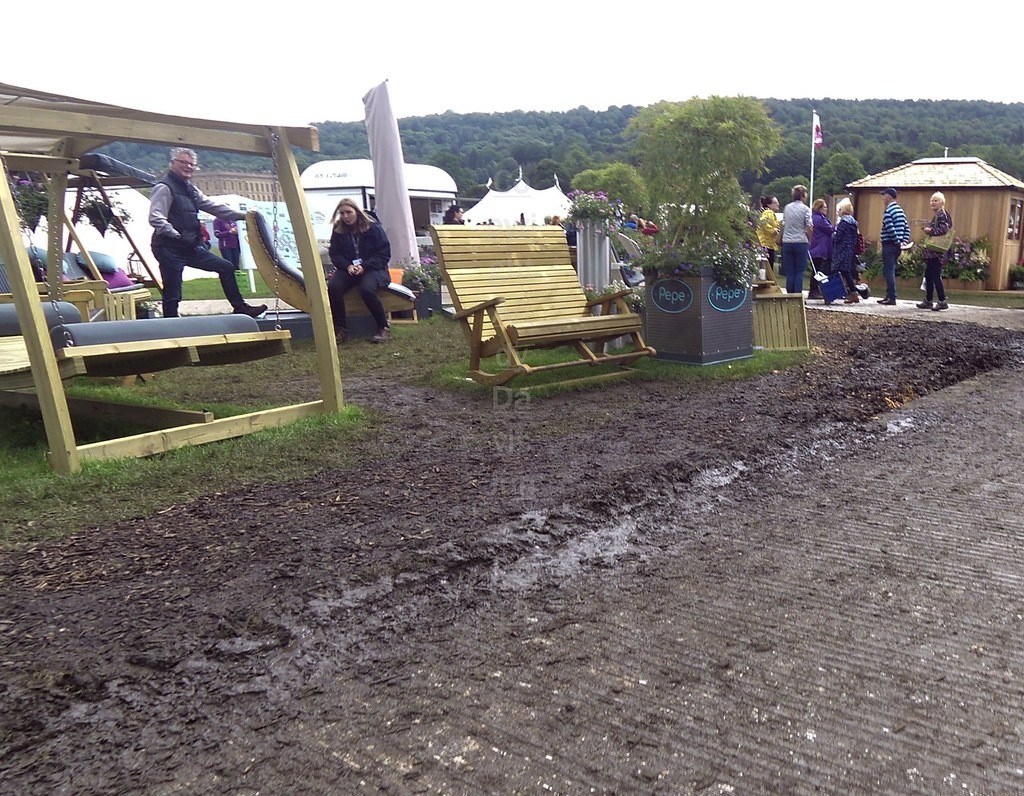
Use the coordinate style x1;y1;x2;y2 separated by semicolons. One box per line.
565;190;623;238
642;232;771;291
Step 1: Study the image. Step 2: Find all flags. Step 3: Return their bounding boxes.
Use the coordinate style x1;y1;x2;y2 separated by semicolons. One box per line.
813;114;823;152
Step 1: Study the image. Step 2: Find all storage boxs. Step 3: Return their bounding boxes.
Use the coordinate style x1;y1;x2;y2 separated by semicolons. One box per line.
819;272;848;303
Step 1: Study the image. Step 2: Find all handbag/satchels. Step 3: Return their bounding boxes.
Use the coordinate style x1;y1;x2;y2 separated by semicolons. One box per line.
857;228;865;254
923;224;955;254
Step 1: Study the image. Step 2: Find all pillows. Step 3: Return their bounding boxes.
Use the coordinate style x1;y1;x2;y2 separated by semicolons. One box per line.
31;244;134;289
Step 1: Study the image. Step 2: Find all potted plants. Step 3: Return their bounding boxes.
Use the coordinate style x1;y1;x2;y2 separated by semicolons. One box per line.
620;94;788;365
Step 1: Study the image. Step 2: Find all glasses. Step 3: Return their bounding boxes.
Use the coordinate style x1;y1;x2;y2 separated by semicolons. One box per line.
174;158;198;167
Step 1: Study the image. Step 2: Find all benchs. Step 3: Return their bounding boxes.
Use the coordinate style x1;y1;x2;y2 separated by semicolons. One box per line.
29;245;152;307
427;222;658;392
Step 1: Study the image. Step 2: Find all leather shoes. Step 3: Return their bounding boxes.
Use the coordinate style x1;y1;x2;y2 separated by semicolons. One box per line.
233;303;268;319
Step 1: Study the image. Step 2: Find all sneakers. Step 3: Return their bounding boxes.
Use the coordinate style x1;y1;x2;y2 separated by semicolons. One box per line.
335;326;348;345
931;299;949;311
370;326;390;344
916;299;933;310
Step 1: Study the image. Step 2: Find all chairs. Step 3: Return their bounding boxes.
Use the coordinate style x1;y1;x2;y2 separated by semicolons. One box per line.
245;210;416;330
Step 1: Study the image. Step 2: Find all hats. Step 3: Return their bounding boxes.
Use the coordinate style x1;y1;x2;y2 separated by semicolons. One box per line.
880;188;897;200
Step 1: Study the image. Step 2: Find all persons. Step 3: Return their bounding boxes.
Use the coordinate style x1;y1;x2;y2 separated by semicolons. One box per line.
477;215;659;238
877;189;910;306
213;217;241;272
781;184;813;294
757;195;780;271
200;221;212;250
328;199;391;345
444;206;463;225
808;199;837;300
825;199;859;304
148;148;267;319
916;191;952;311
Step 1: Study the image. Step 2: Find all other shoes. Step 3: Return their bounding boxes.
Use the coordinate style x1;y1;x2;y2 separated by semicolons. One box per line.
808;291;824;299
845;292;860;304
877;297;896;305
